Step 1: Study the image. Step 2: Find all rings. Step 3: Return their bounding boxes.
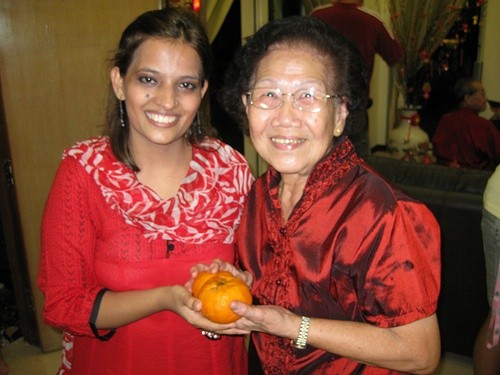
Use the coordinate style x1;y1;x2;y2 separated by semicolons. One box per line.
202;330;220;339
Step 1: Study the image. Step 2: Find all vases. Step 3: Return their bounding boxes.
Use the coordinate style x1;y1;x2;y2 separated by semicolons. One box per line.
390;104;429;151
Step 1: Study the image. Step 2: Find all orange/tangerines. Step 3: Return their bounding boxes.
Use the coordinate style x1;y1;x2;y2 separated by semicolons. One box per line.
192;271;234;298
197;275;253;324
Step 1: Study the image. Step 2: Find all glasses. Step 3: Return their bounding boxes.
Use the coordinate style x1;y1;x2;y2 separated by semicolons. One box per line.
246;87;343;112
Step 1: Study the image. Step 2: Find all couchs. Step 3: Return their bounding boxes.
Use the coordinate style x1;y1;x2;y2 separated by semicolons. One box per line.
365;155;493;357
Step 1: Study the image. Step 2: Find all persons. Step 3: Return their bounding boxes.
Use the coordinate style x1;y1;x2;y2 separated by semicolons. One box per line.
185;18;441;375
44;9;256;375
307;0;403;160
473;166;500;375
433;81;500;166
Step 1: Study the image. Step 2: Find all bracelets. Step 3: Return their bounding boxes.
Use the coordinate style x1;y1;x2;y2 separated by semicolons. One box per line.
291;315;309;349
